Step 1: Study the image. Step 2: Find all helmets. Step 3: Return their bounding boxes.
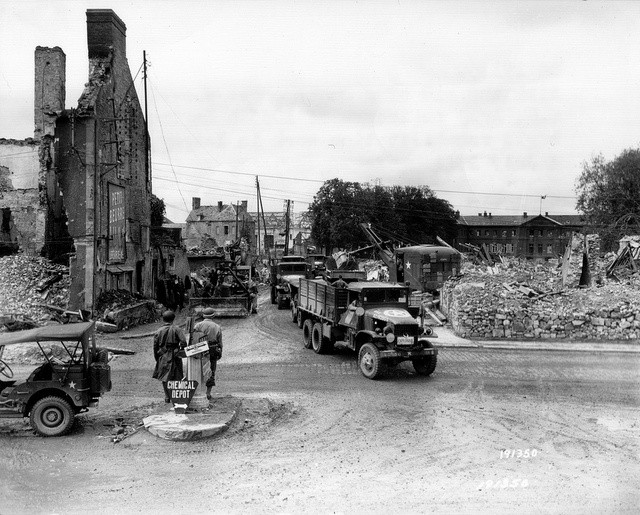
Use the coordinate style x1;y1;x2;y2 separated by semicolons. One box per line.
163;311;175;321
202;307;216;318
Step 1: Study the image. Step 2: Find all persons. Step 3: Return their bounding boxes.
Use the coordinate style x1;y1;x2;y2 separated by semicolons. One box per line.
153;310;187;402
189;307;222;402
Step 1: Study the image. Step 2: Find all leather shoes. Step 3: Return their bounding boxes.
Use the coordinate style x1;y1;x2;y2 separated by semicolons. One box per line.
165;397;170;402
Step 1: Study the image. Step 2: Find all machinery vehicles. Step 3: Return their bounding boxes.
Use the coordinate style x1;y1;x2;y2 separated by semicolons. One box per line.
189;245;258;318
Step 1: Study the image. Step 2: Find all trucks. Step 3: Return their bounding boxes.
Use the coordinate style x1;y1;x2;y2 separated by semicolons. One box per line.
296;278;438;379
269;256;311;311
308;253;326;271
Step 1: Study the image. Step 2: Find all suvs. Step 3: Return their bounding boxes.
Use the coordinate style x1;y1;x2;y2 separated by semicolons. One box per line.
0;321;111;436
